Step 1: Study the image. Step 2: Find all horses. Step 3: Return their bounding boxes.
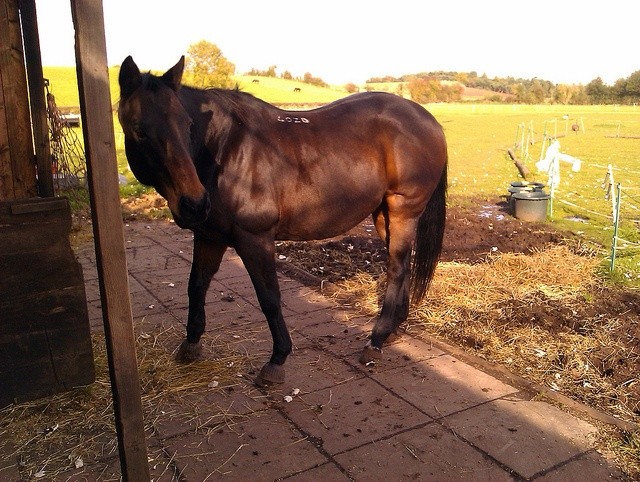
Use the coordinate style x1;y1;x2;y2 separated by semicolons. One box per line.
120;54;448;391
